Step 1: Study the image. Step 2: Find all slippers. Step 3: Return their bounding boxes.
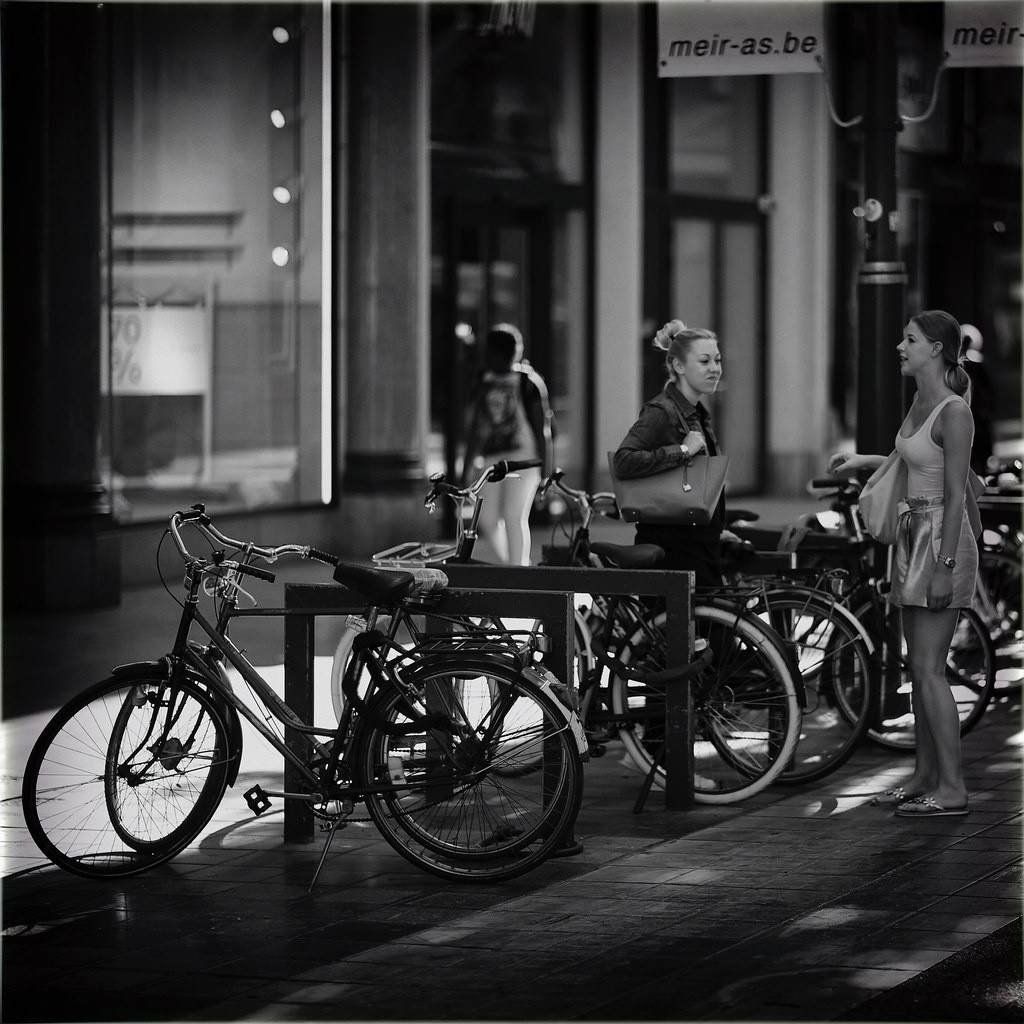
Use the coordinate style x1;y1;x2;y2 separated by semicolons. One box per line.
871;784;914;806
895;792;969;816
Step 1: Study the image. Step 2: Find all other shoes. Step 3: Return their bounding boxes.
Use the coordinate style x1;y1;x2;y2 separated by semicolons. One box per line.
620;745;715;793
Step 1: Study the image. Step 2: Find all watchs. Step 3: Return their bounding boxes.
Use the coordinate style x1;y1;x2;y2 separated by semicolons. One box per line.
679;444;691;460
936;554;956;568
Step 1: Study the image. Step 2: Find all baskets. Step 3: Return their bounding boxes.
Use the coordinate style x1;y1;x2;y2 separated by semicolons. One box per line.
370;542;457;572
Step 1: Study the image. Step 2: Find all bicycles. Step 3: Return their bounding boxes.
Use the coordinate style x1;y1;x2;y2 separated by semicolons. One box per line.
19;461;1024;901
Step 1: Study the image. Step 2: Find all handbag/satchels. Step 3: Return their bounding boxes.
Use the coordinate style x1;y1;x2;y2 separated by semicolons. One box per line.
608;400;730;526
858;447;986;544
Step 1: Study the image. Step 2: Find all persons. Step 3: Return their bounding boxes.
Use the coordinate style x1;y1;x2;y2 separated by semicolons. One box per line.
826;310;979;818
610;317;743;790
451;321;555;566
